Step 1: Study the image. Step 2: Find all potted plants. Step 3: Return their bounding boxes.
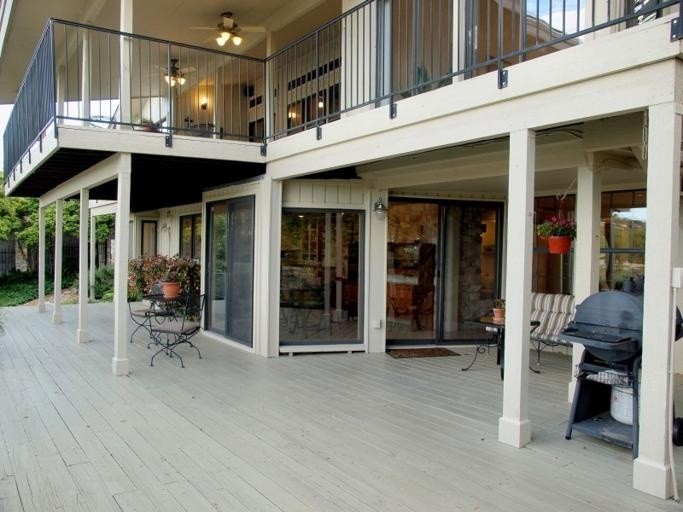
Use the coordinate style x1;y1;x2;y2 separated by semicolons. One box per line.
493;299;505;321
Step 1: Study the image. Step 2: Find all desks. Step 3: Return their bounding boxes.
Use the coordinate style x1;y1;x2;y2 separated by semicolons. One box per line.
463;320;540;381
144;294;194;355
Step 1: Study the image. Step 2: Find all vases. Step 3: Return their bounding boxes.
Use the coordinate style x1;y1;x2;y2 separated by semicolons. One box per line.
163;281;180;299
547;236;570;254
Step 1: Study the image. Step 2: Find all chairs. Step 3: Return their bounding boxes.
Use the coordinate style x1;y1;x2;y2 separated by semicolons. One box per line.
146;294;206;369
128;287;177;344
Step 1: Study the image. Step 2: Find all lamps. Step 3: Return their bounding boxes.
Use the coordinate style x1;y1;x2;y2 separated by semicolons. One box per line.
373;196;389;219
164;74;186;87
215;28;242;46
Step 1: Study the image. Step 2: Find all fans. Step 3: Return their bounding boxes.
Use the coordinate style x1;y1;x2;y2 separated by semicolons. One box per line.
151;59;197;76
190;12;266;42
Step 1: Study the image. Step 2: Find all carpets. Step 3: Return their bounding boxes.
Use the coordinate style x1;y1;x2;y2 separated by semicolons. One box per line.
386;348;461;359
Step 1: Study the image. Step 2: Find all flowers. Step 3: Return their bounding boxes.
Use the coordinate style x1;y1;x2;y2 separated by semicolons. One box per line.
152;253;195;283
537;216;577;238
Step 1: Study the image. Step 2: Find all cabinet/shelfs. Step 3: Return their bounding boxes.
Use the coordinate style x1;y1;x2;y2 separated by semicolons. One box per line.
388;241;436;324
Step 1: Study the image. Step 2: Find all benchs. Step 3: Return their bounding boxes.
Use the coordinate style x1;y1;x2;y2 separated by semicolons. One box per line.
485;292;574;366
